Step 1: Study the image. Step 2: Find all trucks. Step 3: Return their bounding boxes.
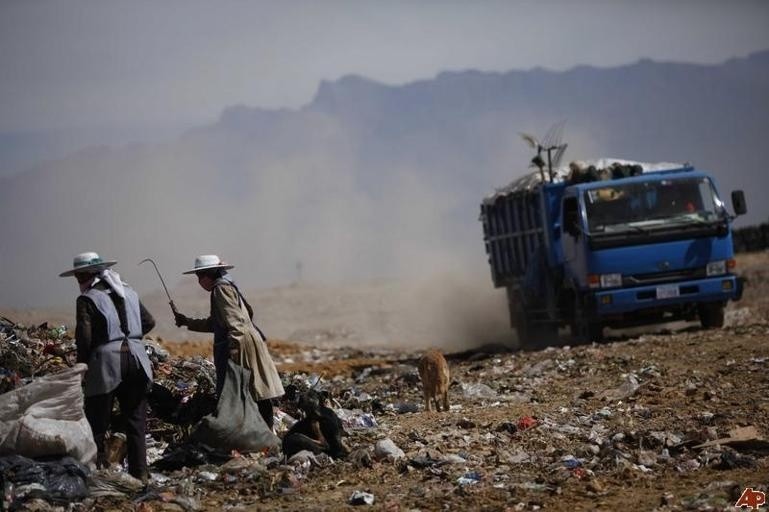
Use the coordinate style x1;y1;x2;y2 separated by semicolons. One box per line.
477;160;749;347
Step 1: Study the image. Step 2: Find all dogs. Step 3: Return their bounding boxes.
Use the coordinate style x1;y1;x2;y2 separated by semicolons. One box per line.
281;405;347;458
417;348;451;414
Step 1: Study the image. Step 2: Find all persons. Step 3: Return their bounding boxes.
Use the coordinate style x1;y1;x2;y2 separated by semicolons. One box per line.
58;252;154;494
174;255;286;433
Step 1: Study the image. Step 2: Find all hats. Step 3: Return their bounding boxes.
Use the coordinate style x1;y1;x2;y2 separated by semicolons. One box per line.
182;255;235;274
59;252;118;277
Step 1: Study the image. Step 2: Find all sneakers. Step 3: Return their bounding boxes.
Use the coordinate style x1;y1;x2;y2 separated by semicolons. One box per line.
128;472;147;485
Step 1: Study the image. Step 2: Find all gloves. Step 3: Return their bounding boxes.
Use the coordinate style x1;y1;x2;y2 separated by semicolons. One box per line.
173;313;190;326
228;349;239;360
